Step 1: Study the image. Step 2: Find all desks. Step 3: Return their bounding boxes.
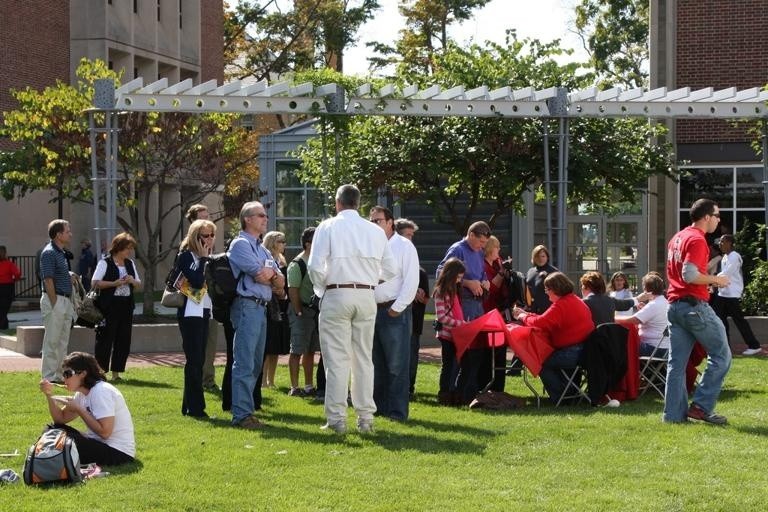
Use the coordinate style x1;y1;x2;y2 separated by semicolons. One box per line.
449;317;639;411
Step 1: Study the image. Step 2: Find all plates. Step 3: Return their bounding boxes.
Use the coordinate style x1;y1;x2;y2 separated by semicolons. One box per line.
50;394;74;402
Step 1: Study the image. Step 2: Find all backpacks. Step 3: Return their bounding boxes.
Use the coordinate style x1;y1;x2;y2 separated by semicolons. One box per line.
204;232;245;306
23;427;83;483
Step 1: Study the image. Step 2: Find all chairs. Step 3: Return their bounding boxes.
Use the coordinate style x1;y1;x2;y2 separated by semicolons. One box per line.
551;322;632;417
637;323;702;402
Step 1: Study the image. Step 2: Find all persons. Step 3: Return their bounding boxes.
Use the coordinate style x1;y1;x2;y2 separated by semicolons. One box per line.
0;243;23;329
91;232;142;381
305;184;400;432
708;224;763;357
662;197;734;426
174;202;429;427
38;351;137;482
38;219;75;385
78;237;107;300
431;221;670;408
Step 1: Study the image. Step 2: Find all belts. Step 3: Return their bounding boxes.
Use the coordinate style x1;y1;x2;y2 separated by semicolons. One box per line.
676;298;703;302
327;283;377;292
42;289;71;299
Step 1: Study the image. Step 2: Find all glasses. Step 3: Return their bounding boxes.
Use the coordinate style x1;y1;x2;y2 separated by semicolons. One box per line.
62;370;82;377
199;232;215;238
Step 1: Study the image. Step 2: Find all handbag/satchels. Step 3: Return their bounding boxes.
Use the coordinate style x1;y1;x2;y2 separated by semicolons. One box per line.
160;288;185;308
77;291;104;324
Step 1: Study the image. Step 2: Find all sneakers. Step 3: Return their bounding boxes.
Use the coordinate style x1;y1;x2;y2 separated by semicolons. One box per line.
203;383;324;405
686;402;728;425
238;417;263;430
320;420;347;432
357;418;375;434
742;348;762;355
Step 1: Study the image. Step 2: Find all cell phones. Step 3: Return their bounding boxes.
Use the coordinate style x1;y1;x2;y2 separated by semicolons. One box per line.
197;235;205;246
